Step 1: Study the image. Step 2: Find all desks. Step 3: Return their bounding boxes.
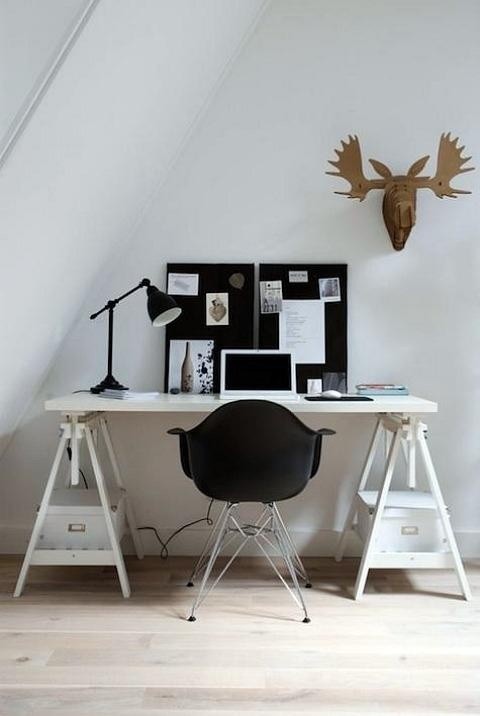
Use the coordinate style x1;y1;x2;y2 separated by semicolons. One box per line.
9;391;472;599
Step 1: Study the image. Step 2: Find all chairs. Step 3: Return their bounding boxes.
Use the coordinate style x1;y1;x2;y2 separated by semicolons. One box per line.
166;400;337;623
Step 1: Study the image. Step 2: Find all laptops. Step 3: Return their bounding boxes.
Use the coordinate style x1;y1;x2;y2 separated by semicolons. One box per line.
219;350;297;400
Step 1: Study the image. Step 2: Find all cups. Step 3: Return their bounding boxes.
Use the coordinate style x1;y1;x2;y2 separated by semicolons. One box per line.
308;379;322;393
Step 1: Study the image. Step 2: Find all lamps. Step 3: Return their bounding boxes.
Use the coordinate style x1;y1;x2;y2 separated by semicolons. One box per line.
89;279;181;395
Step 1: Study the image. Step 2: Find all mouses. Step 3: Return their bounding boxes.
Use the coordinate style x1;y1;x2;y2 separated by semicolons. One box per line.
322;390;342;399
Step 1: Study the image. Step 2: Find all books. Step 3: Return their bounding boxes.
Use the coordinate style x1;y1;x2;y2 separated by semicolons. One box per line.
98;389;160;401
357;390;409;396
356;384;407;390
